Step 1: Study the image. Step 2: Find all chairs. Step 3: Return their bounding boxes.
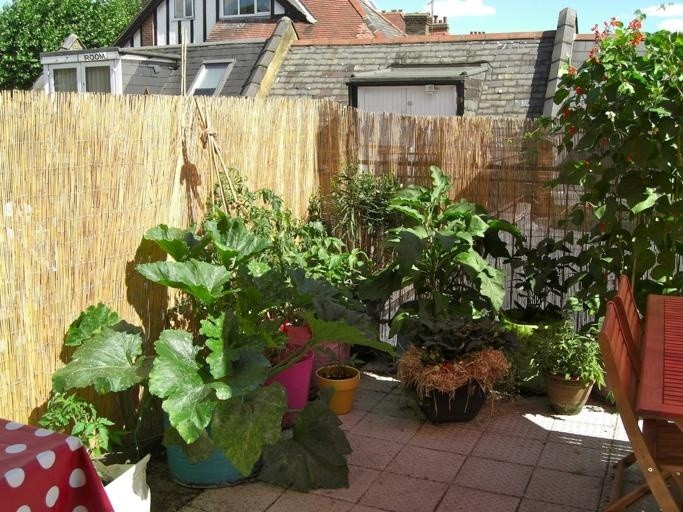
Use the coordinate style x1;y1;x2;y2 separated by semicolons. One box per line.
596;274;683;512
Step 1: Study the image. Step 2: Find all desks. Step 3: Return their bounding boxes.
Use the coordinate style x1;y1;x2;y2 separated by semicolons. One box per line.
0;418;80;512
